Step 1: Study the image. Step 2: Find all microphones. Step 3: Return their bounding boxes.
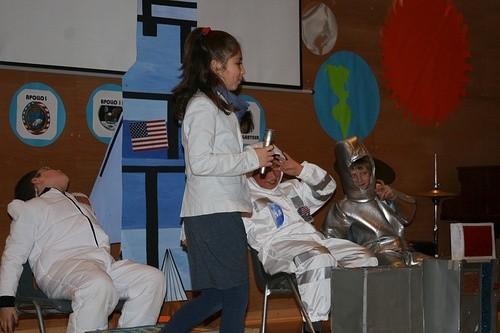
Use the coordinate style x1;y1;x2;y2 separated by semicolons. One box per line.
259;128;275;175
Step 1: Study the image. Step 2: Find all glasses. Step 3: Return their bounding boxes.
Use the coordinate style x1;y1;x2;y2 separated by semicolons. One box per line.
34;166;50;178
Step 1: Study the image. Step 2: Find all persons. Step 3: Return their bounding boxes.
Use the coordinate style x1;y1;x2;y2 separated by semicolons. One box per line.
180;143;378;333
323;137;437;268
0;166;166;333
158;27;276;333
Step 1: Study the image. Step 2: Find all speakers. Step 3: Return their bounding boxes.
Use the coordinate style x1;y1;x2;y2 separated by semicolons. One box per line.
332;266;422;333
